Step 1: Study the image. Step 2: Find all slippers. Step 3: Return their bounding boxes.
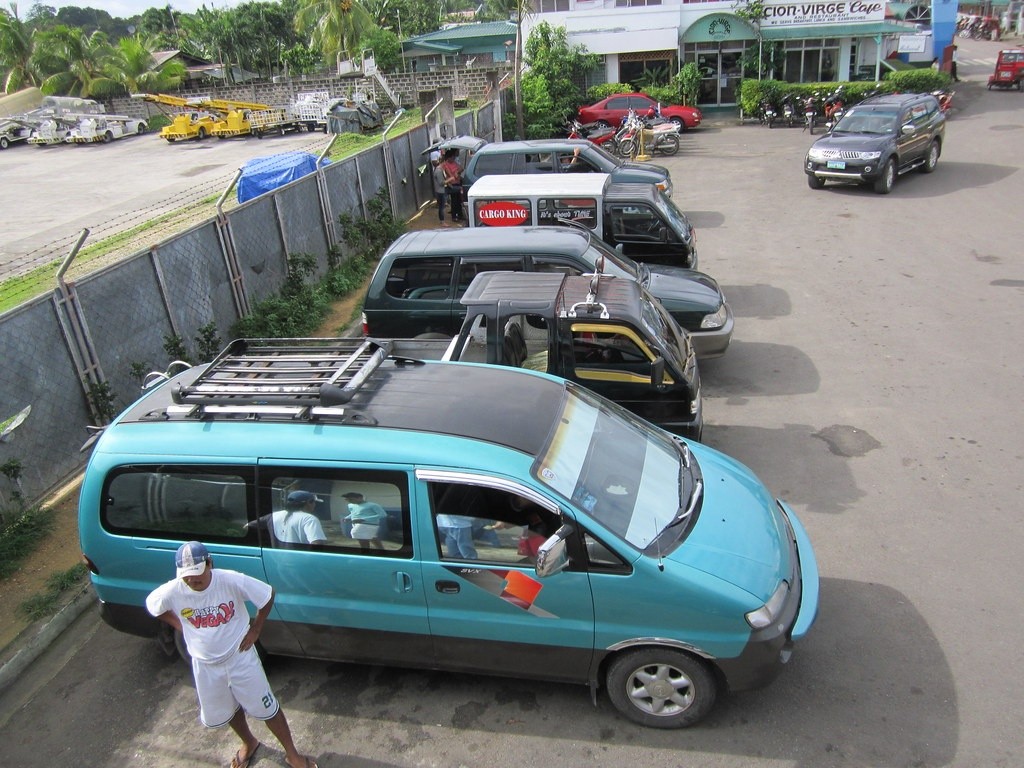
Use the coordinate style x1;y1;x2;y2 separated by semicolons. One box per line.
285;755;318;768
231;741;260;768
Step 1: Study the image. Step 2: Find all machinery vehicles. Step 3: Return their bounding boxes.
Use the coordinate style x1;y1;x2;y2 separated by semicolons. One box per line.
142;93;223;142
198;99;292;139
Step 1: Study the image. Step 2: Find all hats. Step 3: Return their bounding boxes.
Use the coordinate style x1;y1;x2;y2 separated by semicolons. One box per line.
287;492;324;505
174;541;208;580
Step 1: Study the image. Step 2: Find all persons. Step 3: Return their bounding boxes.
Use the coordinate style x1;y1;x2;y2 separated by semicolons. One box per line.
341;492;387;548
431;140;442;171
561;148;579;164
931;57;939;72
433;148;464;226
437;514;478;559
145;540;318;768
952;46;961;82
243;491;327;544
483;520;545;557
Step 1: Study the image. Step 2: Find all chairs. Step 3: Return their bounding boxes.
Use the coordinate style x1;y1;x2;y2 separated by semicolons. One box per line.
502;322;548;372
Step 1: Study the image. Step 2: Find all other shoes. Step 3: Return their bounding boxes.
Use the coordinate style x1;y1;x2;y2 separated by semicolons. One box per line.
441;223;450;227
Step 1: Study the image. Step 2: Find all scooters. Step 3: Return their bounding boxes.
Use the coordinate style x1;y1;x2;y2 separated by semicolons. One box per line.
988;48;1024;92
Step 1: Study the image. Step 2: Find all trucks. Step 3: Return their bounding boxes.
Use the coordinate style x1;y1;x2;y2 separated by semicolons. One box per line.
441;270;704;443
468;173;698;272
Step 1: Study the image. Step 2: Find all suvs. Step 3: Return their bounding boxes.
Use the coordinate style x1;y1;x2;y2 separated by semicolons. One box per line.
80;338;819;730
803;92;945;194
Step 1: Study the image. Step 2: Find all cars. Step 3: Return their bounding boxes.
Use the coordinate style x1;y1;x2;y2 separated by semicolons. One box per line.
577;93;702;134
421;134;673;221
362;220;735;357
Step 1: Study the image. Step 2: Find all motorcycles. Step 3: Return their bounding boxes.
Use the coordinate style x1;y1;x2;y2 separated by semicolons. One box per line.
956;15;1005;41
821;89;844;127
864;84;952;119
757;97;777;128
615;107;681;159
796;92;820;135
780;96;801;128
561;119;616;155
